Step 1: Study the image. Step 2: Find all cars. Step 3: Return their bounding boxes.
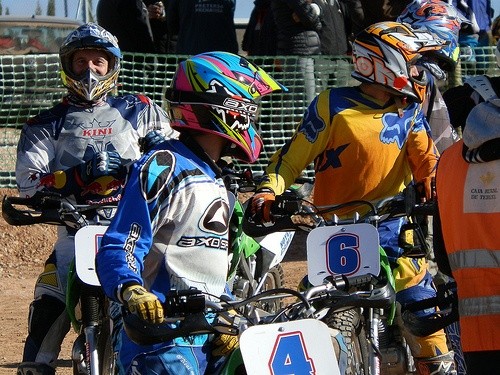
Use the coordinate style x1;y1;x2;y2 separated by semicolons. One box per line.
0;15;86;124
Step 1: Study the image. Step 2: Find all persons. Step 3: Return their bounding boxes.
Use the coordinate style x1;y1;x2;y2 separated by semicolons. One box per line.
94;0;500;375
15;22;180;375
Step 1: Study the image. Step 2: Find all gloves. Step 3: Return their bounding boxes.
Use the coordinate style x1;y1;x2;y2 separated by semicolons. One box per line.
414;175;438;203
123;285;166;325
75;150;133;186
212;308;244;356
246;187;279;230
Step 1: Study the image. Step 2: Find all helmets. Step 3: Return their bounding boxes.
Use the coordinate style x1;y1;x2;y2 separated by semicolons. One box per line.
396;0;473;70
164;50;289;163
351;21;451;103
58;21;121;101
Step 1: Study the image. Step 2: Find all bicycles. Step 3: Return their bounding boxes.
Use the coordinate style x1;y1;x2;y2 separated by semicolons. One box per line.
2;168;459;375
